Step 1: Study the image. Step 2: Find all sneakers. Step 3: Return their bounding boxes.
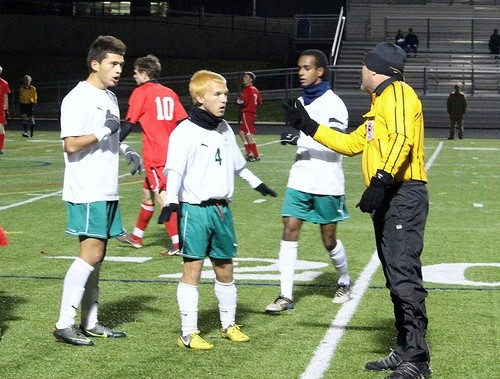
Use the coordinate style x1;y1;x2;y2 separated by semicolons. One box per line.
52;324;94;346
265;295;294;311
117;233;142;248
80;321;125;338
384;361;433;379
178;330;214;350
222;324;250;342
334;279;354;303
161;246;181;256
366;348;403;370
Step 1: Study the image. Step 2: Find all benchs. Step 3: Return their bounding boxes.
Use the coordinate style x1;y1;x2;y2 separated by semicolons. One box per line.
323;0;500;133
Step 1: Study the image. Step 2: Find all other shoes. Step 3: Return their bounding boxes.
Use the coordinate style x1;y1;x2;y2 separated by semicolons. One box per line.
448;137;454;140
31;134;33;137
23;133;29;137
0;149;3;154
246;156;252;161
249;157;260;161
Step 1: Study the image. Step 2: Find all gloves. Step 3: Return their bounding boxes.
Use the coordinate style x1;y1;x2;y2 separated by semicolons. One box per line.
282;98;317;135
5;110;9;119
256;183;277;197
158;203;182;224
356;169;393;213
125;147;141;175
281;132;299;145
235;97;240;105
104;110;120;136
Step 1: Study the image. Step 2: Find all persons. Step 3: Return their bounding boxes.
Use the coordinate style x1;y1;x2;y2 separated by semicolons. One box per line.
53;37;144;347
119;56;189;256
236;72;262;162
263;49;352;314
282;42;431;379
489;29;500;59
447;85;467;139
18;75;38;138
395;28;419;58
0;66;11;154
159;71;277;352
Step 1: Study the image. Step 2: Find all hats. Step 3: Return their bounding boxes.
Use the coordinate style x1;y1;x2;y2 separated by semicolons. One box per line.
363;42;406;77
